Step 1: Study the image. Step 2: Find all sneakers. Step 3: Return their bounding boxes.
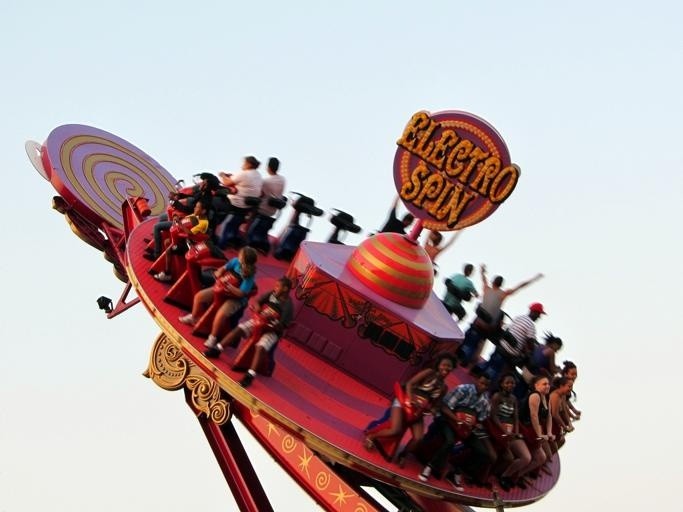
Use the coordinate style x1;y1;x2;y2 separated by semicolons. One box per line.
144;252;157;262
152;271;171;281
363;434;375;451
202;335;221;359
445;469;493;492
177;314;197;325
419;463;434;482
239;372;253;387
497;463;552;492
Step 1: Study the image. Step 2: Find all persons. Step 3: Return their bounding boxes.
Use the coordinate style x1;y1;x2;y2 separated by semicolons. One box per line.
143;157;293;387
381;195;461;263
365;261;583;494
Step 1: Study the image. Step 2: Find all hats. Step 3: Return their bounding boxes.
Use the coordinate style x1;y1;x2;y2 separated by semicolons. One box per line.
530;302;547;315
